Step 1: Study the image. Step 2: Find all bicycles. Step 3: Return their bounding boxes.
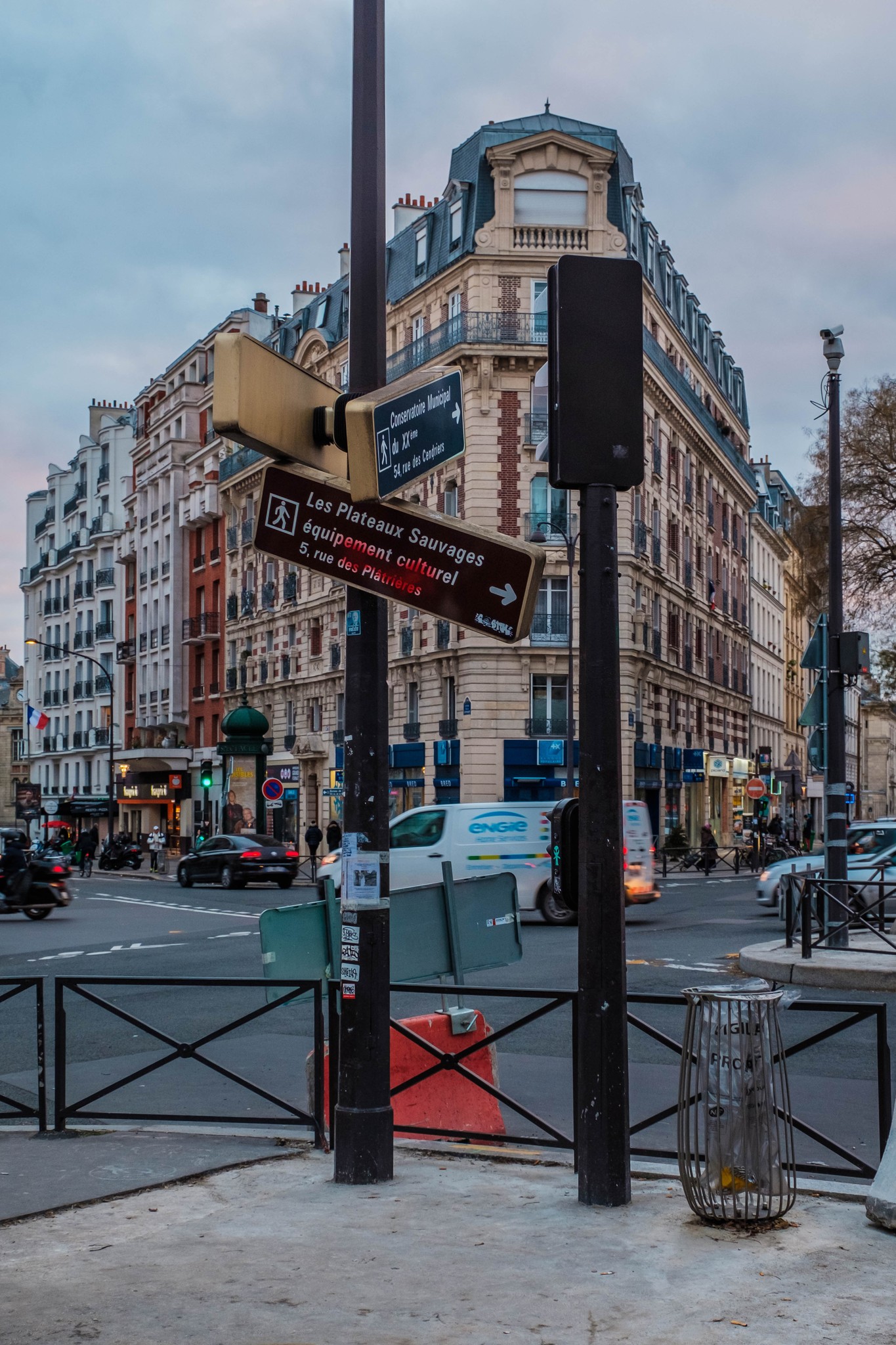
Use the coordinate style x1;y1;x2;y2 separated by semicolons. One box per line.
78;849;96;877
731;829;803;870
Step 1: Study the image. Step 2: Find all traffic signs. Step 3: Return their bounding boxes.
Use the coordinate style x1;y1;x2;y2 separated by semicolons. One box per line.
343;360;468;505
253;458;544;644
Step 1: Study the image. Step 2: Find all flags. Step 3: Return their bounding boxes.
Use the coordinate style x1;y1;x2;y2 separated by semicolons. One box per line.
708;581;716;610
27;705;49;729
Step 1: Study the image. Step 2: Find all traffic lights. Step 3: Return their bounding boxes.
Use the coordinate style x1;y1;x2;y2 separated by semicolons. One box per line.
200;757;213;788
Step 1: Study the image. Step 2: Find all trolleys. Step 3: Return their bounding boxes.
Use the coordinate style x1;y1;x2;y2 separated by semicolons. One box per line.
680;848;708;872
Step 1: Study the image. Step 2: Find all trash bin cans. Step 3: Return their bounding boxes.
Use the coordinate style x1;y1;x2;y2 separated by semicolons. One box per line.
678;980;801;1224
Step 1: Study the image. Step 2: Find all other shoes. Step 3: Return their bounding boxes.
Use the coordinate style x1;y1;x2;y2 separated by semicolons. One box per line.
804;850;810;852
694;864;713;873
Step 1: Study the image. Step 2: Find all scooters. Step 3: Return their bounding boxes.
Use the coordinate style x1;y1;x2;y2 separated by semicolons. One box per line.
28;839;54;861
98;832;144;871
0;855;73;920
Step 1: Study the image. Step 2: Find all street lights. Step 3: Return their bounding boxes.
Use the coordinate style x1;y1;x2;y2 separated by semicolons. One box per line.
25;639;131;848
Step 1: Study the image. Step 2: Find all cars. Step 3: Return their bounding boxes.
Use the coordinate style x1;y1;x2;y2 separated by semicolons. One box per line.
756;818;896;921
177;832;300;888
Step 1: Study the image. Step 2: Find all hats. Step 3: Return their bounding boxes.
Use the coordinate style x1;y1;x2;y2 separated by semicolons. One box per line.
153;825;159;830
81;828;87;833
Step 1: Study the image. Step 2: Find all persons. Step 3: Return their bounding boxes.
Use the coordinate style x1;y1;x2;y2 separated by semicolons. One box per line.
90;823;99;860
235;808;256;833
326;820;342;852
147;825;166;873
21;791;38;809
227;790;243;834
195;827;207;849
305;820;323;868
76;828;99;869
58;826;69;841
700;824;719;873
768;814;812;852
0;839;28;901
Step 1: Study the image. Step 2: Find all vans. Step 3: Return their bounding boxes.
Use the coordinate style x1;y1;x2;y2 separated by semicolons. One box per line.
316;799;660;925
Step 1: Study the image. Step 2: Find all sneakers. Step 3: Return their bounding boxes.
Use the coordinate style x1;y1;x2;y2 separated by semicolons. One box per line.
150;869;158;873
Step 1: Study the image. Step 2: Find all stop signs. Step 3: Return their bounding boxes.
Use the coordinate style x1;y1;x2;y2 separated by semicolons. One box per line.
262;778;284;801
746;778;766;799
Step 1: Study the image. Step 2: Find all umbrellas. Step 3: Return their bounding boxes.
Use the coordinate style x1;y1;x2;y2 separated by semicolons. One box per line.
42;821;71;829
